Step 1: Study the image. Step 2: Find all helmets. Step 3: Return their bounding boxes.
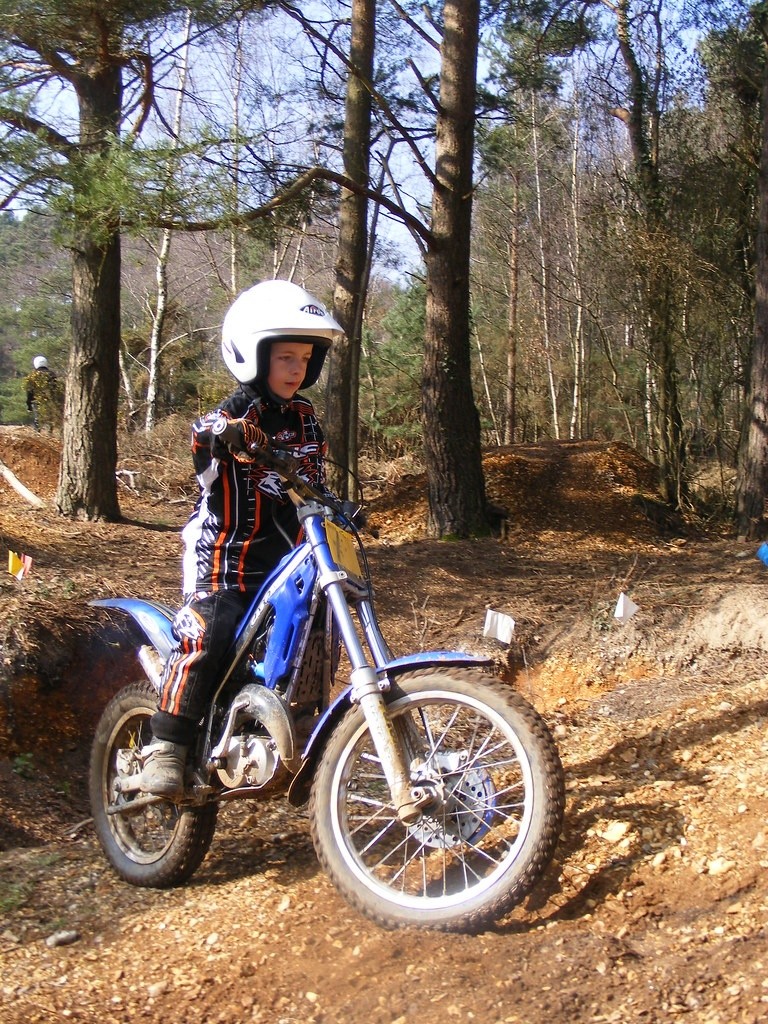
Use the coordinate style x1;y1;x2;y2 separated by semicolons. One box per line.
32;357;48;370
221;281;347;386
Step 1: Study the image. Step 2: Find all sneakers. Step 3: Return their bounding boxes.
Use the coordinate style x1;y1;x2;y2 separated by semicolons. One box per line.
141;739;190;800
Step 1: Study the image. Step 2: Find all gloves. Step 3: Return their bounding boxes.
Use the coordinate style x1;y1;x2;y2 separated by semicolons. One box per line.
219;417;268;465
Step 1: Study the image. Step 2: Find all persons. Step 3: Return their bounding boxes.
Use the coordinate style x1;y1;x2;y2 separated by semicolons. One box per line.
26;355;57;433
139;280;346;801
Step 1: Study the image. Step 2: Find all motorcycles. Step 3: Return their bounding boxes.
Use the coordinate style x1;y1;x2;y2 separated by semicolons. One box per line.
83;415;569;936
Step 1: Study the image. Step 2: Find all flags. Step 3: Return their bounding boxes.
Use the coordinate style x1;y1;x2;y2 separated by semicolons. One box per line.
483;610;515;645
8;550;32;580
756;543;768;566
615;592;639;624
